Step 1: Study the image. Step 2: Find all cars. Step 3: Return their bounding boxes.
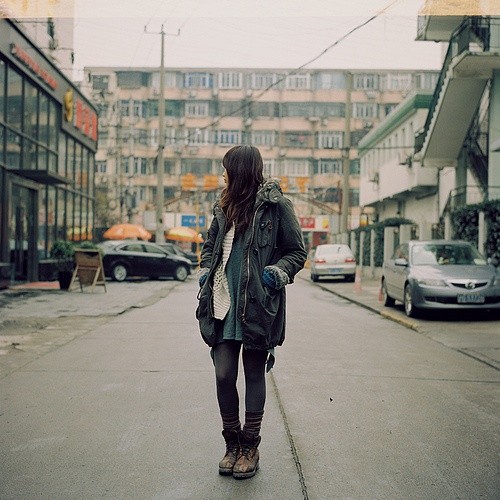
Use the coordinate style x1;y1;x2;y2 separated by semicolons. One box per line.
101;240;193;281
10;224;67;260
310;244;356;283
155;243;199;269
380;240;500;317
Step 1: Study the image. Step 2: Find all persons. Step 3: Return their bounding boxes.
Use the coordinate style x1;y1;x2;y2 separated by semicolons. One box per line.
195;145;306;480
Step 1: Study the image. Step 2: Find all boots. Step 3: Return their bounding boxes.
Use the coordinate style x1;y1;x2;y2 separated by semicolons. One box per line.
233;434;261;479
219;428;241;475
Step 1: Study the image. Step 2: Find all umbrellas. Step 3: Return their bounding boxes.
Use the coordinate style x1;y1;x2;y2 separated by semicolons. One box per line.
102;223;152;240
166;226;204;243
